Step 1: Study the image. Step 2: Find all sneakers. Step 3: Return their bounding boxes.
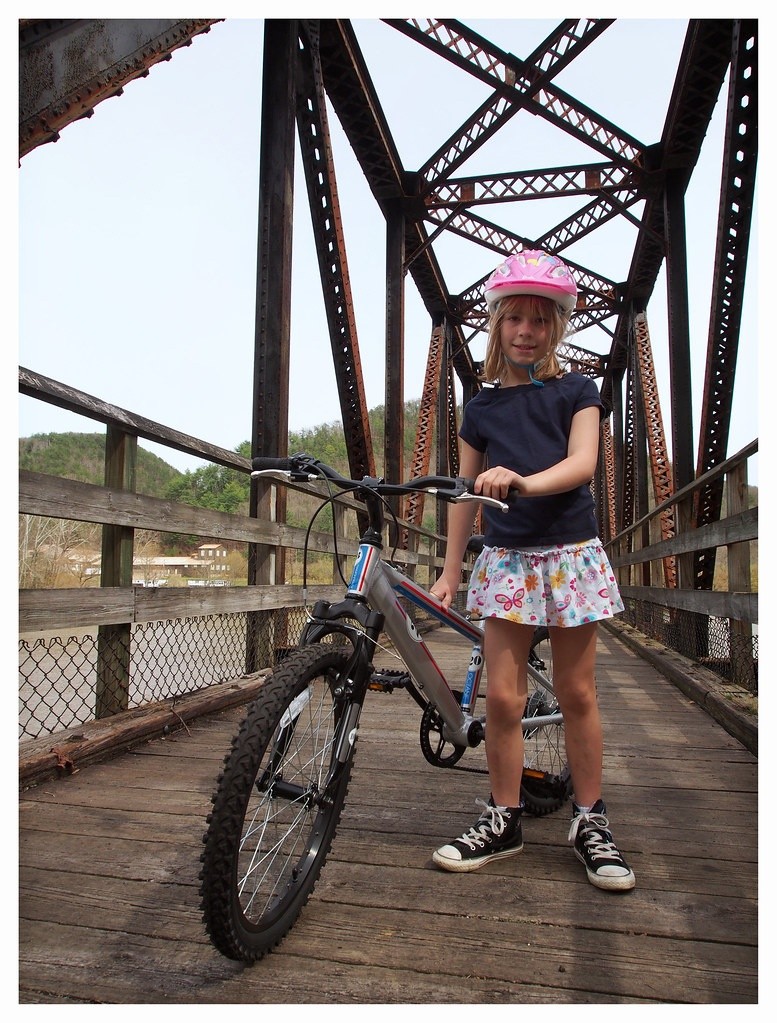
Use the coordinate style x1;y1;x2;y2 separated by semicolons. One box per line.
572;799;636;891
433;790;525;872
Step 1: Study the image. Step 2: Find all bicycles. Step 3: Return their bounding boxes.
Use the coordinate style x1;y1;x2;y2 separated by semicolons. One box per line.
200;454;572;973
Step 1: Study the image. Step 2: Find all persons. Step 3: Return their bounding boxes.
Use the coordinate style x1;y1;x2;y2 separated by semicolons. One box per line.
429;250;643;892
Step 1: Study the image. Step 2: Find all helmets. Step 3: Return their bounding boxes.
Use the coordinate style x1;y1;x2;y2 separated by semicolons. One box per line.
485;250;578;314
